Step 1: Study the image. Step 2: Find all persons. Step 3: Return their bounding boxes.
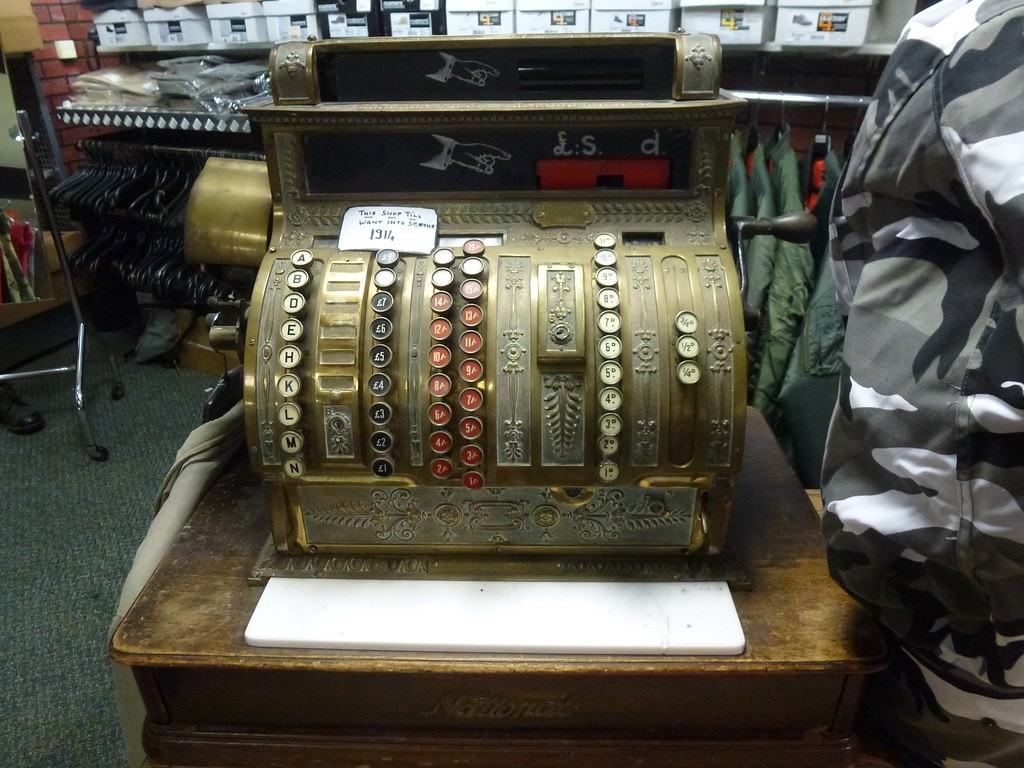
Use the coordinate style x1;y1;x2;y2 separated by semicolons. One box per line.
822;1;1022;768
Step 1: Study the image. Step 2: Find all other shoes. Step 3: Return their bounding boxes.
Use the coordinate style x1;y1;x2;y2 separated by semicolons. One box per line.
0;382;45;436
